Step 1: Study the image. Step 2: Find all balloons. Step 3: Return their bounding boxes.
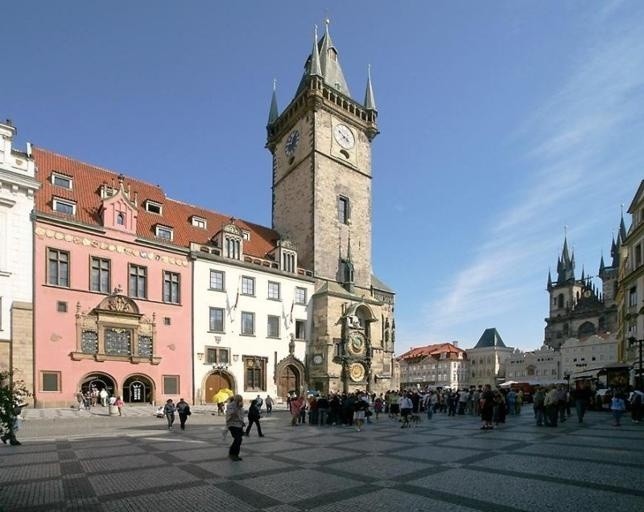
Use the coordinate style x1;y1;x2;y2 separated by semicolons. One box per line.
284;130;299;157
333;123;355;150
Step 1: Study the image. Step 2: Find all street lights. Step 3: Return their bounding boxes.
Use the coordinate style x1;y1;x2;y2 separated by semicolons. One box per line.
365;408;373;416
630;392;641;407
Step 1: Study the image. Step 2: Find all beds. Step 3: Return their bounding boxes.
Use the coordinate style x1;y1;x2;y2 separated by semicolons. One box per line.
218;388;233;398
211;392;229;405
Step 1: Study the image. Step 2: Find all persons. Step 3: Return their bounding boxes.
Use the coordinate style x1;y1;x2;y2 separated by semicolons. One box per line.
106;394;116;414
75;383;108;413
162;399;176;429
224;394;246;462
176;398;191;431
113;396;124;417
265;395;274;415
217;403;225;417
155;406;164;419
255;395;263;413
245;400;265;438
